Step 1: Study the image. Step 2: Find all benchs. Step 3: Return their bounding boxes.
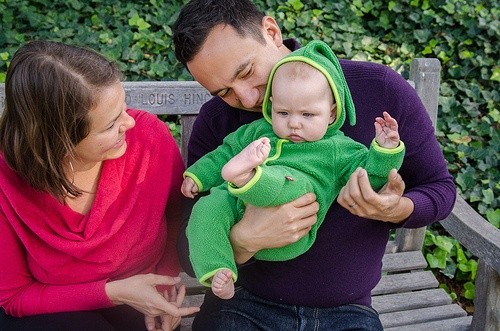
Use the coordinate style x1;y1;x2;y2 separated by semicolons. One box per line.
1;58;500;331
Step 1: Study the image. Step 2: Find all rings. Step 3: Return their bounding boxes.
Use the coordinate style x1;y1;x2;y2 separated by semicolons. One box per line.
350;201;357;208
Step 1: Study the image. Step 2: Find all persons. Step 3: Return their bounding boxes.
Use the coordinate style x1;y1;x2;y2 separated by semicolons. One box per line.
0;40;200;331
172;0;457;331
180;52;404;300
